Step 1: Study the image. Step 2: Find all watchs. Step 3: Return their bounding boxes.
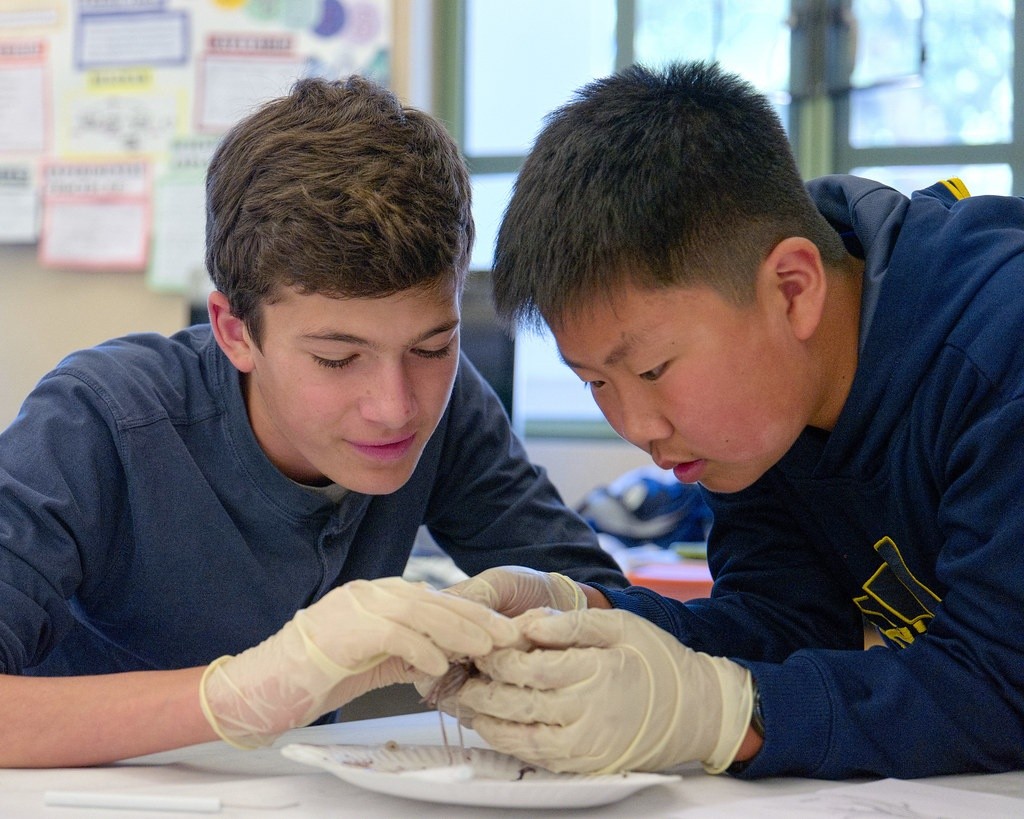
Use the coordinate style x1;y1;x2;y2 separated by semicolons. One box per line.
751;681;766;738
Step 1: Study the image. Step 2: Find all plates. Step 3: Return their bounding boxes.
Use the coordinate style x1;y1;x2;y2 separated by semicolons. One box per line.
283;742;684;809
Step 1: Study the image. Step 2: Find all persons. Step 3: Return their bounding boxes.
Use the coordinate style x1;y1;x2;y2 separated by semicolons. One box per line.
418;61;1024;781
0;75;632;772
